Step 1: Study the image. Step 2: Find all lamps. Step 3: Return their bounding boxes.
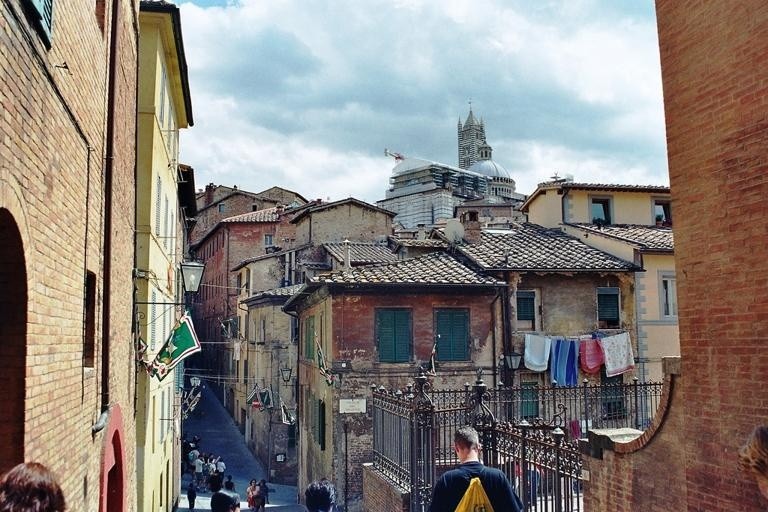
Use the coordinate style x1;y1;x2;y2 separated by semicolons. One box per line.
179;376;201;392
132;250;206;305
280;363;301;386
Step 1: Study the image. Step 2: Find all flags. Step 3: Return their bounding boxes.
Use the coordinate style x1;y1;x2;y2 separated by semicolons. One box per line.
315;334;336;388
146;307;202;382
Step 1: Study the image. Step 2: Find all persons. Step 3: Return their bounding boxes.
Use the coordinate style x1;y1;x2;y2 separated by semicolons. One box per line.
183;434;269;512
305;478;337;512
739;426;768;501
427;426;525;512
0;462;65;512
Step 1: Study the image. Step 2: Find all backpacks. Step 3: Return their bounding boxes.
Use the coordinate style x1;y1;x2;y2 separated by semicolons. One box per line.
454;466;496;512
